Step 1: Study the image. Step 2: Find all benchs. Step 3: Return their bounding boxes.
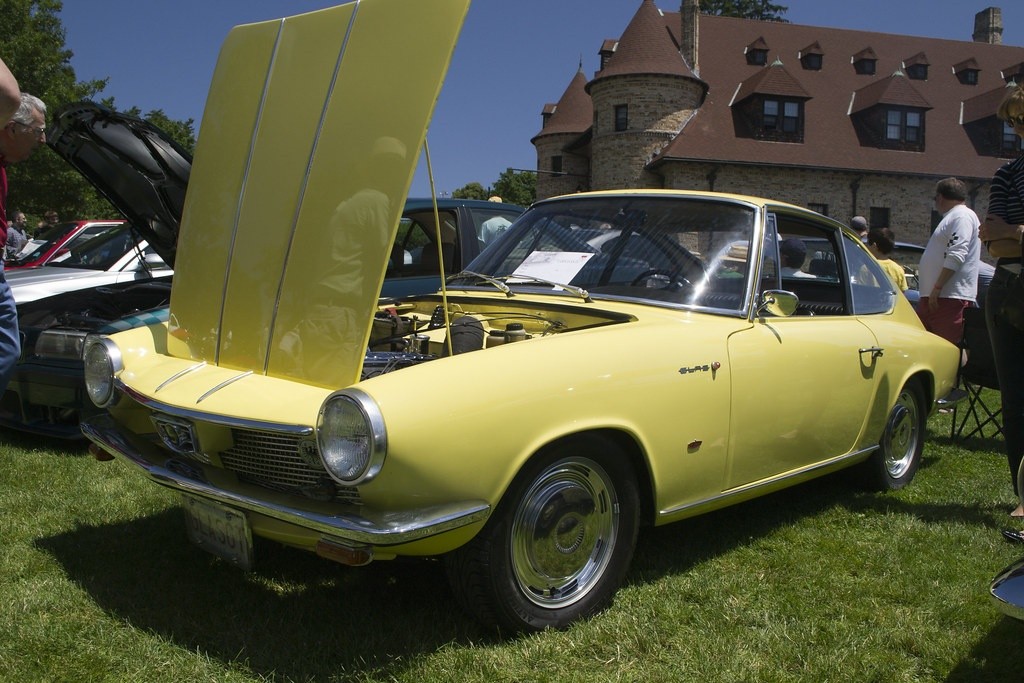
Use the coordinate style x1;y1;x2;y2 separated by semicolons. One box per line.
701;277;877;316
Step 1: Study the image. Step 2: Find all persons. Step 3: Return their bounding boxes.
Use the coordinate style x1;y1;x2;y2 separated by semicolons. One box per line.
480;196;908;294
977;81;1023;542
0;54;48;370
918;177;981;343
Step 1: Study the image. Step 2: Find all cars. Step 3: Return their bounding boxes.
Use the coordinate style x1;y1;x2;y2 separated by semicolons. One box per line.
0;100;601;439
80;1;960;632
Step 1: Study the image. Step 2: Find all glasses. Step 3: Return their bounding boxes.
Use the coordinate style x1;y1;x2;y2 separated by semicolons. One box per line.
4;119;47;136
1007;114;1024;128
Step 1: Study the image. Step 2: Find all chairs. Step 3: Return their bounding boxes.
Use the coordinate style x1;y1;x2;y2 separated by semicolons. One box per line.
950;306;1006;445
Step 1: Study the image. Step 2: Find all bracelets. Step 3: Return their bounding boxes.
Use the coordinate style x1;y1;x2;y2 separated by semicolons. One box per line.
935;284;942;288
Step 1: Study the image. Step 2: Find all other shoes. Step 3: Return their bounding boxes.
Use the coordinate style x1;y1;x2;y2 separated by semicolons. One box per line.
1001;529;1024;543
